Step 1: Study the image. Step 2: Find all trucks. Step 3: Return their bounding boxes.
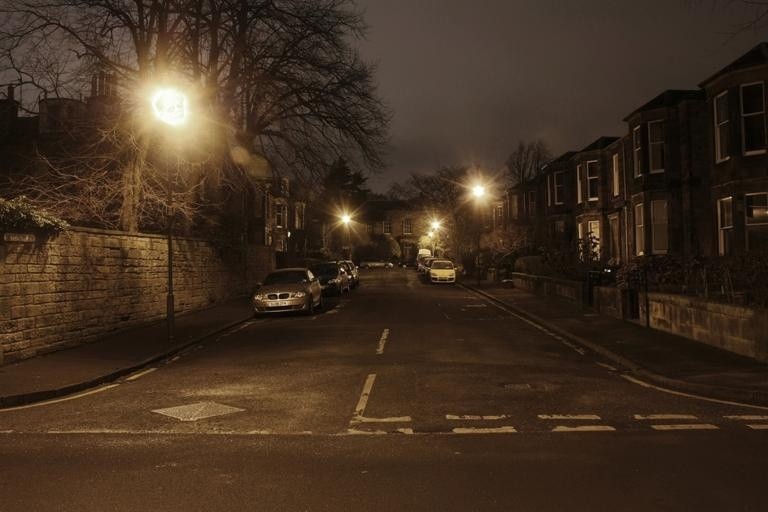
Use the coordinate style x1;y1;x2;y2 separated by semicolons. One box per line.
417;248;431;261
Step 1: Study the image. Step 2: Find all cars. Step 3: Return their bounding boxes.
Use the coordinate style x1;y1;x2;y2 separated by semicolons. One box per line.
419;256;456;284
309;260;359;297
252;268;324;315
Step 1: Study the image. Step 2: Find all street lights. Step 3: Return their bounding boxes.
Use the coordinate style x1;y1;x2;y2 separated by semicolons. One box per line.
151;88;187;332
473;186;485;288
